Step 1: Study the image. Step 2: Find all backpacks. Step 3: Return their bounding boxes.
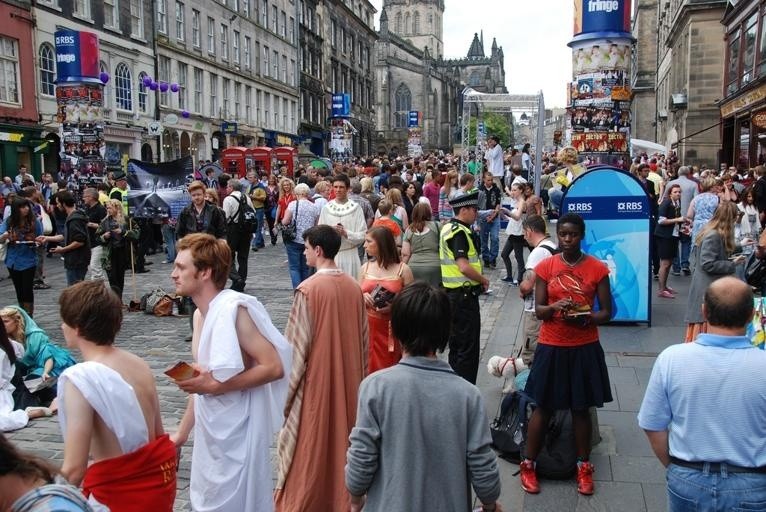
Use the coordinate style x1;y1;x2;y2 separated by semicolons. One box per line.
488;390;538;477
228;194;258;233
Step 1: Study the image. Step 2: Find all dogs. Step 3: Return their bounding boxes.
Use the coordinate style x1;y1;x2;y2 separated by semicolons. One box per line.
487;355;529;394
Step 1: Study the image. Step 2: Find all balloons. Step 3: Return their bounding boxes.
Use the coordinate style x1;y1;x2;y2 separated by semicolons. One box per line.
142;75;152;87
171;84;179;92
159;82;168;92
150;82;158;90
183;110;190;118
100;72;109;84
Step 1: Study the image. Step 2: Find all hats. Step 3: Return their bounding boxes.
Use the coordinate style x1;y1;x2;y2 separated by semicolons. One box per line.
448;190;479;208
112;171;126;180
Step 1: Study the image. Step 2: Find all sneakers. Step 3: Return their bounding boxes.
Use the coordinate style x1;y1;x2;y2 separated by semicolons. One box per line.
251;245;258;252
490;258;497;268
230;279;246;291
671;271;681;276
161;260;172;263
521;459;540;493
259;243;265;248
657;288;678;298
484;257;490;267
682;267;691;274
511;280;520;285
272;238;277;245
502;276;513;282
654;274;659;280
576;461;594;497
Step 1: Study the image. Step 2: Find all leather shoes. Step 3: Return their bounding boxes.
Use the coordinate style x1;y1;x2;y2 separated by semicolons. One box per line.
137;269;150;273
26;407;52;418
33;281;48;290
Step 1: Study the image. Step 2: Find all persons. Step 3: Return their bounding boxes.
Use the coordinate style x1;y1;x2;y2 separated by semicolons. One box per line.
336;120;343;127
0;135;766;510
572;43;631;130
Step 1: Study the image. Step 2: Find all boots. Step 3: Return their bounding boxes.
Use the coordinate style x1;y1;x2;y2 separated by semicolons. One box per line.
184;304;196;342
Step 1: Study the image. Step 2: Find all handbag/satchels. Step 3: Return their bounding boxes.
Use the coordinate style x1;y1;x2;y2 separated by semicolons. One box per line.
0;232;8;262
41;214;53;235
281;219;297;244
153;294;183;317
746;246;766;289
539;188;548;197
272;223;281;236
270;206;277;219
146;289;167;315
140;294;152;310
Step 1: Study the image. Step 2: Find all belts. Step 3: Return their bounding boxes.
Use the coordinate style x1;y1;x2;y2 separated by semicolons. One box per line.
668;454;762;475
